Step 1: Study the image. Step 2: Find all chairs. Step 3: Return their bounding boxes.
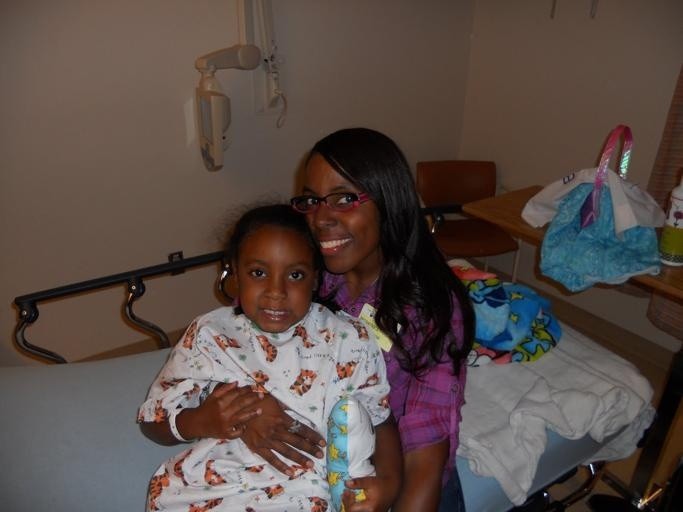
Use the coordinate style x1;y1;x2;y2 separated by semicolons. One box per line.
412;157;521;276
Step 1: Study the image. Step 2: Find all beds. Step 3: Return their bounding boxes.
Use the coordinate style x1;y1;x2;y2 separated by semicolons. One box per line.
1;255;655;511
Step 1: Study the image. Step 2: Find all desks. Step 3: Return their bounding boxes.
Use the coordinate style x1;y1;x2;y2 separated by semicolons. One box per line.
464;184;683;511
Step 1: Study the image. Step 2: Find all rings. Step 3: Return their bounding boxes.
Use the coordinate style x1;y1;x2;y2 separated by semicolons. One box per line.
287;418;301;434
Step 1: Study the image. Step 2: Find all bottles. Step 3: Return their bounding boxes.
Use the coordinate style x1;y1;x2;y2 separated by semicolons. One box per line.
659;172;683;267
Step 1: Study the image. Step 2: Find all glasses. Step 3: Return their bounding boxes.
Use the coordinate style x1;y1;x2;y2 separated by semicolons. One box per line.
289;190;368;212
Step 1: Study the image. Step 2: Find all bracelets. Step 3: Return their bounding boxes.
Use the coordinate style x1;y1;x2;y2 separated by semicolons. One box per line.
167;410;196;445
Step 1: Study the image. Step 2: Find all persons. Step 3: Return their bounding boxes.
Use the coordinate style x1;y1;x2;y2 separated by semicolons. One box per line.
208;124;469;511
134;202;405;511
